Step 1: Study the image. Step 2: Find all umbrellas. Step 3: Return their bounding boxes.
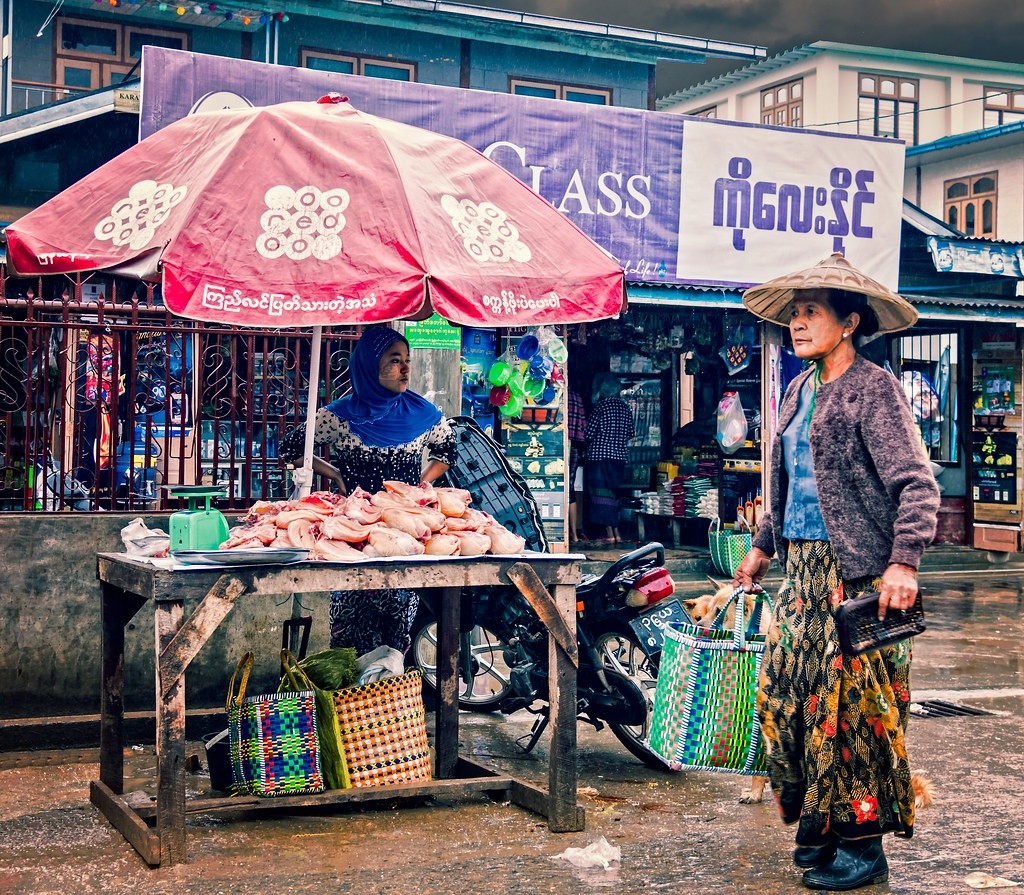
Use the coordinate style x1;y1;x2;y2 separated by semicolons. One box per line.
4;93;628;666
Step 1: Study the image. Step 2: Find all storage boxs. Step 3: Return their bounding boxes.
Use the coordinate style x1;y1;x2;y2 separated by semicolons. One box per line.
624;445;660;484
193;352;336;499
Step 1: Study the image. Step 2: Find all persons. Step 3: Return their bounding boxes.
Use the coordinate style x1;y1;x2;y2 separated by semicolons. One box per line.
985;373;1011;396
568;390;587;547
277;325;456;657
733;252;941;891
585;372;635;542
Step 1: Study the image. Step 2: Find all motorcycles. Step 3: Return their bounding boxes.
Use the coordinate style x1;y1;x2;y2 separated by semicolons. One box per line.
405;413;700;771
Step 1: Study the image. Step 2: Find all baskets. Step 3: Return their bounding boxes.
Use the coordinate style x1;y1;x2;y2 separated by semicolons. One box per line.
226;648;326;798
313;667;433;791
708;514;753;580
643;581;771;776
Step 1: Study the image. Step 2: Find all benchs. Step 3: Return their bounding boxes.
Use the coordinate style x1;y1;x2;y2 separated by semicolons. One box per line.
633;511;689;548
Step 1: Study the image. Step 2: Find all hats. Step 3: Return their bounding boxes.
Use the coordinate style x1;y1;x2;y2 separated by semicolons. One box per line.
742;252;920;335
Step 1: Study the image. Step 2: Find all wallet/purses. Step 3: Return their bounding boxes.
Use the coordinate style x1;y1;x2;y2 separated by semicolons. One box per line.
833;587;926;656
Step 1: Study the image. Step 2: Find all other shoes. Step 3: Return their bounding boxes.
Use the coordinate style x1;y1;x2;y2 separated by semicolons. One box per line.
615;536;623;543
597;537;617;544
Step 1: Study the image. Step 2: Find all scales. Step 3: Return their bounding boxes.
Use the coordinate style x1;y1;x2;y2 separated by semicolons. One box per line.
163;484;230;553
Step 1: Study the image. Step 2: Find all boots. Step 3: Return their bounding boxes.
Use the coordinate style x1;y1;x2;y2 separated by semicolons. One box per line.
801;836;889;891
793;833;838;867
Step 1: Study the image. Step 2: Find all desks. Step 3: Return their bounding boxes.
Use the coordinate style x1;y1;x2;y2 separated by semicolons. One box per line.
90;547;586;869
